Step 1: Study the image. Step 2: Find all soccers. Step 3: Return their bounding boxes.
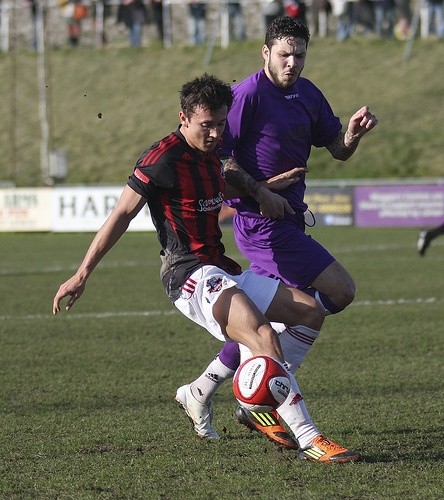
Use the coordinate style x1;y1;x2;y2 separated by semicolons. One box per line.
231;354;291;413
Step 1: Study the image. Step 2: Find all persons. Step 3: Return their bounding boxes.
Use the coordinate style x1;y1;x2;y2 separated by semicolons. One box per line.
53;74;361;463
174;15;378;440
28;0;443;50
417;220;444;259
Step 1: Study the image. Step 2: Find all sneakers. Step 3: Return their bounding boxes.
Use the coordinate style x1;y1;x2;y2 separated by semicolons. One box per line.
298;435;361;463
236;403;299;450
175;384;220;442
